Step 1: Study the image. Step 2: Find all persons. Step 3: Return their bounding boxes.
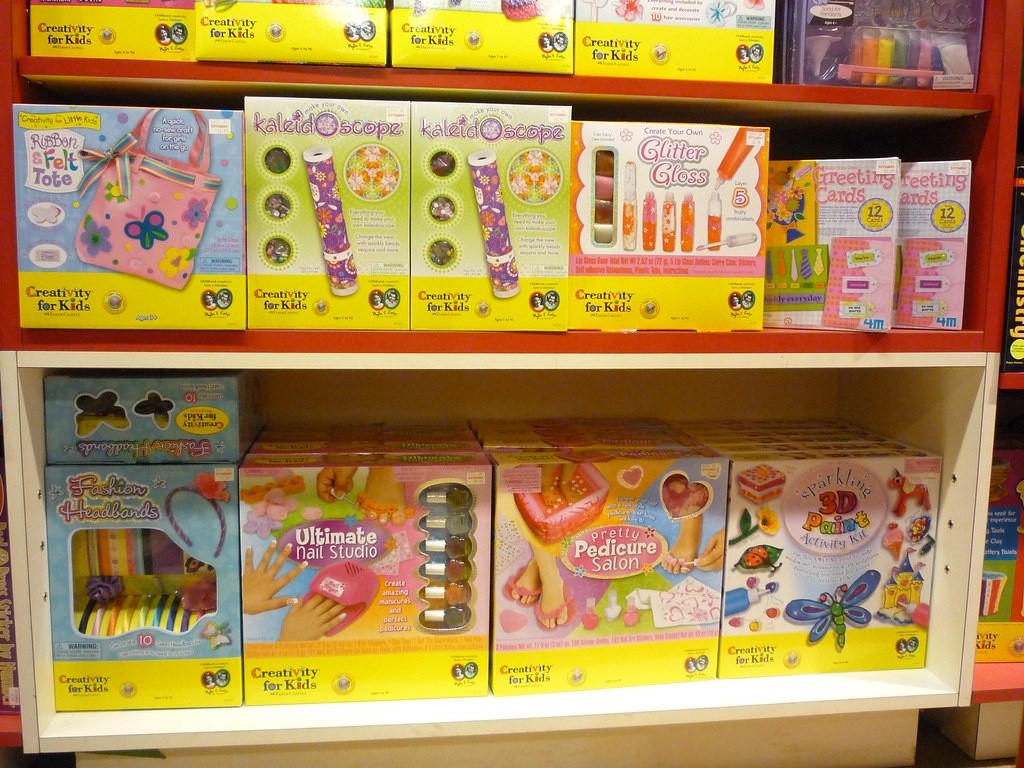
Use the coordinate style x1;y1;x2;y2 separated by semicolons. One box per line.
663;514;725;574
242;540;346;643
316;466;405;526
503;540;572;630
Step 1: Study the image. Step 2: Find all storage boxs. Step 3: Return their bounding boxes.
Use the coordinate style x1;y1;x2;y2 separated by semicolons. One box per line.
41;371;1024;715
12;0;985;337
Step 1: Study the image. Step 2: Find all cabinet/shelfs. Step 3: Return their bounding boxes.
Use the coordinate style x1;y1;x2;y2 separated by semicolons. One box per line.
0;0;1024;356
0;351;1000;754
